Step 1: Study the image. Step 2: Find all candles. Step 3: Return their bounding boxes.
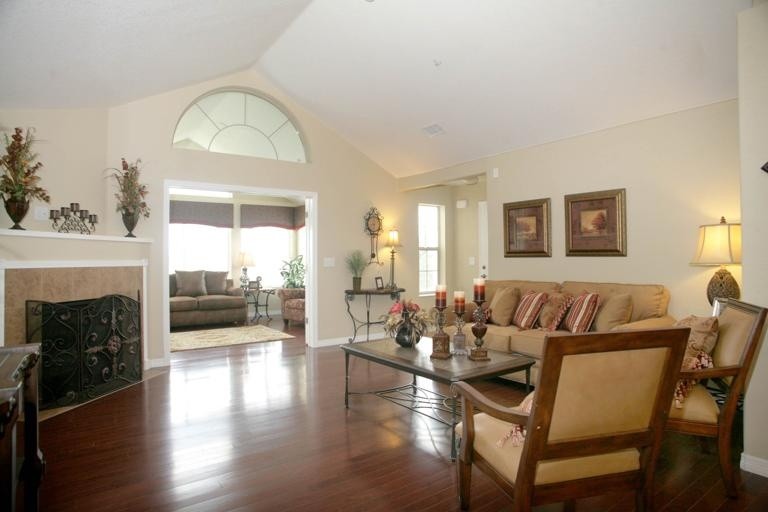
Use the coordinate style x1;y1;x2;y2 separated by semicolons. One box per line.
435;289;447;310
474;278;484;301
453;290;464;312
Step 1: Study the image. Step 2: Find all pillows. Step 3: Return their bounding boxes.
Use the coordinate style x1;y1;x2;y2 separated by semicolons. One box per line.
513;287;549;330
175;271;207;296
673;336;714;410
563;287;600;334
487;287;520;325
535;291;574;331
678;315;721;356
205;271;229;294
499;388;537;453
592;290;633;334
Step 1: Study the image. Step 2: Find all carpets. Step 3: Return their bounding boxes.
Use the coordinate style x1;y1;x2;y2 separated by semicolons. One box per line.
169;323;296;354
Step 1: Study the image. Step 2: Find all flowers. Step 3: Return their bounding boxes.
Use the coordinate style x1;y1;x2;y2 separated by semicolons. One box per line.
103;158;151;222
1;128;51;204
382;302;434;343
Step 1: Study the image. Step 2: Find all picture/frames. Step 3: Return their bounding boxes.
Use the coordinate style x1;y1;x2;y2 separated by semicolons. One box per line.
374;277;383;289
501;196;553;258
562;188;629;258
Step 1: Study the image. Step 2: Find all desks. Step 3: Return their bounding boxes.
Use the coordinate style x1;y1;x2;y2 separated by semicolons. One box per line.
343;287;404;344
1;343;48;511
243;289;276;321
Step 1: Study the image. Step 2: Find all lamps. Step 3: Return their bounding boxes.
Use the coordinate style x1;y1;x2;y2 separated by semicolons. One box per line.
384;229;404;290
689;217;744;306
234;253;253;284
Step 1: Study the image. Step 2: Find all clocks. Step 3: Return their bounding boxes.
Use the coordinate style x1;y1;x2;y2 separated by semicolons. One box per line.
364;208;387;266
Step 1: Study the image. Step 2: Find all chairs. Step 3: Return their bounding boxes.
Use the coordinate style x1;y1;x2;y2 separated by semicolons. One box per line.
279;288;304;325
662;295;768;500
447;327;693;510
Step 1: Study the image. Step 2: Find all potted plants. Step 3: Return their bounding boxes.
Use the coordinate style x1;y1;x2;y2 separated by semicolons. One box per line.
343;251;368;290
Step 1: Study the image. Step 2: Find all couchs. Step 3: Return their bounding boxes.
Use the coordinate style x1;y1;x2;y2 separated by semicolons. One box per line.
169;270;248;329
432;279;677;390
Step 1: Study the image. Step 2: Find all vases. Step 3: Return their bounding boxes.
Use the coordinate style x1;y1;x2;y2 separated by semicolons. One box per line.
397;326;420;347
122;212;138;234
4;198;32;231
